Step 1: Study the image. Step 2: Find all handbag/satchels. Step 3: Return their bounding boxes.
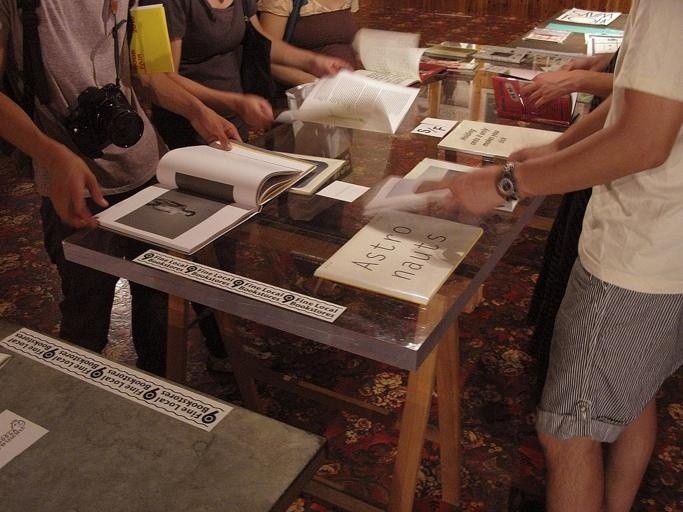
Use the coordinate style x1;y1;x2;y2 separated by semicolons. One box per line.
239;13;273;96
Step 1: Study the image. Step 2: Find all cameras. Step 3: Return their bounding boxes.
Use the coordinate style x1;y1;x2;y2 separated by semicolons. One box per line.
60;83;144;159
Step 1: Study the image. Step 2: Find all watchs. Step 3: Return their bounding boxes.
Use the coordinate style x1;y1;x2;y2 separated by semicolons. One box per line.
496;163;520;204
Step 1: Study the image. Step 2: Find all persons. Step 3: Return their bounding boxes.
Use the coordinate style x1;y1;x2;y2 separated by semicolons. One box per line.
257;0;359;86
1;1;244;377
414;0;683;511
521;49;618;400
151;0;354;373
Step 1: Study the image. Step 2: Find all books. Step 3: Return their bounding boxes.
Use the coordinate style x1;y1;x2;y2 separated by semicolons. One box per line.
439;119;564;161
92;139;318;256
286;152;349;195
362;155;523;220
491;75;579;125
350;27;530;89
313;212;484;306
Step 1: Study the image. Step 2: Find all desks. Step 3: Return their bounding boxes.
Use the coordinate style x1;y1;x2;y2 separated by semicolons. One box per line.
60;37;615;512
0;312;335;512
504;6;631;109
